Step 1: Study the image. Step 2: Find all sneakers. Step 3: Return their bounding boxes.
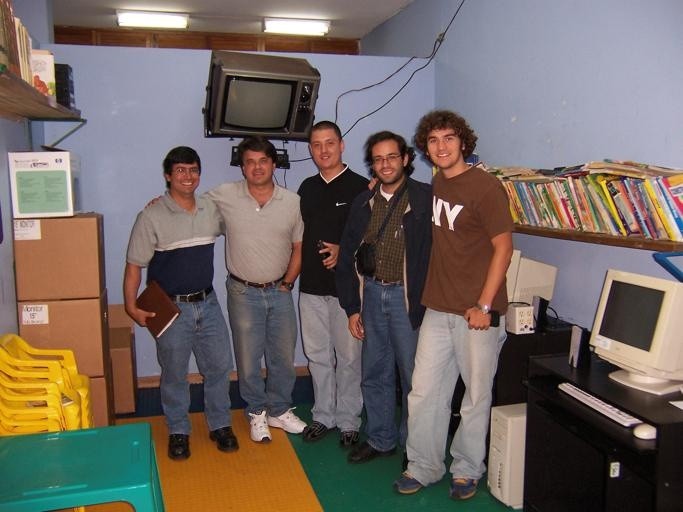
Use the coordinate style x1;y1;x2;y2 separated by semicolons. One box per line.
250;407;396;463
393;471;423;494
450;478;479;499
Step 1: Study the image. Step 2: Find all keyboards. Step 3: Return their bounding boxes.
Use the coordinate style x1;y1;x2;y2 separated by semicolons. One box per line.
560;382;643;427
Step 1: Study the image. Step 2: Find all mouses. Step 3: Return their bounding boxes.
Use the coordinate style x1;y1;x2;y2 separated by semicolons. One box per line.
634;422;657;440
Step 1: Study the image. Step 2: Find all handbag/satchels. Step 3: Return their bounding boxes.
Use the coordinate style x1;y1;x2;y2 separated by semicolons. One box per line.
355;242;376;275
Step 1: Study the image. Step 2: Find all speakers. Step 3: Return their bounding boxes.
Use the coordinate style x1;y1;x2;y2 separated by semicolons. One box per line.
569;325;591;369
532;296;548;329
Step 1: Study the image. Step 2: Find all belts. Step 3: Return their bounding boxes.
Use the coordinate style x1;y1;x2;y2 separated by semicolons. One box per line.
171;286;213;302
229;273;285;287
372;276;403;286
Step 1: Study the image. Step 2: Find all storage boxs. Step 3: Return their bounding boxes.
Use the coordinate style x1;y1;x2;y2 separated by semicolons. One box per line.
104;303;140;414
16;298;111;377
11;212;106;300
5;146;83;219
89;377;115;427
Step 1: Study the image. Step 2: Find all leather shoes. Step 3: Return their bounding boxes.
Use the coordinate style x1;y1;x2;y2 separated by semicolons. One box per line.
209;426;239;451
168;434;190;459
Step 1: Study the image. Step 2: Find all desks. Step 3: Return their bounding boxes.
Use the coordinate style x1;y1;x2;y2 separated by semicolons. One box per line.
0;423;164;511
522;346;682;510
496;312;592;405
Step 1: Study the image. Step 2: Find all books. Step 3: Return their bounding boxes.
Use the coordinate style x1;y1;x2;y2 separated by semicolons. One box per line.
433;159;681;241
132;278;180;342
0;0;58;106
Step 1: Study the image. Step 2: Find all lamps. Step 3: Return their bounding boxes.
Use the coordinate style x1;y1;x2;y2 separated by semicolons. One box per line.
261;16;330;39
113;8;190;32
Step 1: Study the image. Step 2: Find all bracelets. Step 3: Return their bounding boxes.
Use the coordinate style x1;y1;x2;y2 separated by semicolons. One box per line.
281;280;294;288
474;302;494;315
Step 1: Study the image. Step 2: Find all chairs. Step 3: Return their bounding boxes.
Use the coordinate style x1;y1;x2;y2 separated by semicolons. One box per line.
0;334;89;512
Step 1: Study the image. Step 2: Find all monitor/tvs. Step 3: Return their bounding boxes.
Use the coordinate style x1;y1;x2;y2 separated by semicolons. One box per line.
586;269;682;394
505;249;559;311
205;49;322;140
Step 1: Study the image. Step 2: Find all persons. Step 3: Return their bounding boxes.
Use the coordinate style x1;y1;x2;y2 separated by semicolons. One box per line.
121;145;238;460
334;128;433;472
296;120;371;449
392;109;516;498
144;135;309;444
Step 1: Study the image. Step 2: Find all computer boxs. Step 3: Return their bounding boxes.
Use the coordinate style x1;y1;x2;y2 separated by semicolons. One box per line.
488;403;526;508
